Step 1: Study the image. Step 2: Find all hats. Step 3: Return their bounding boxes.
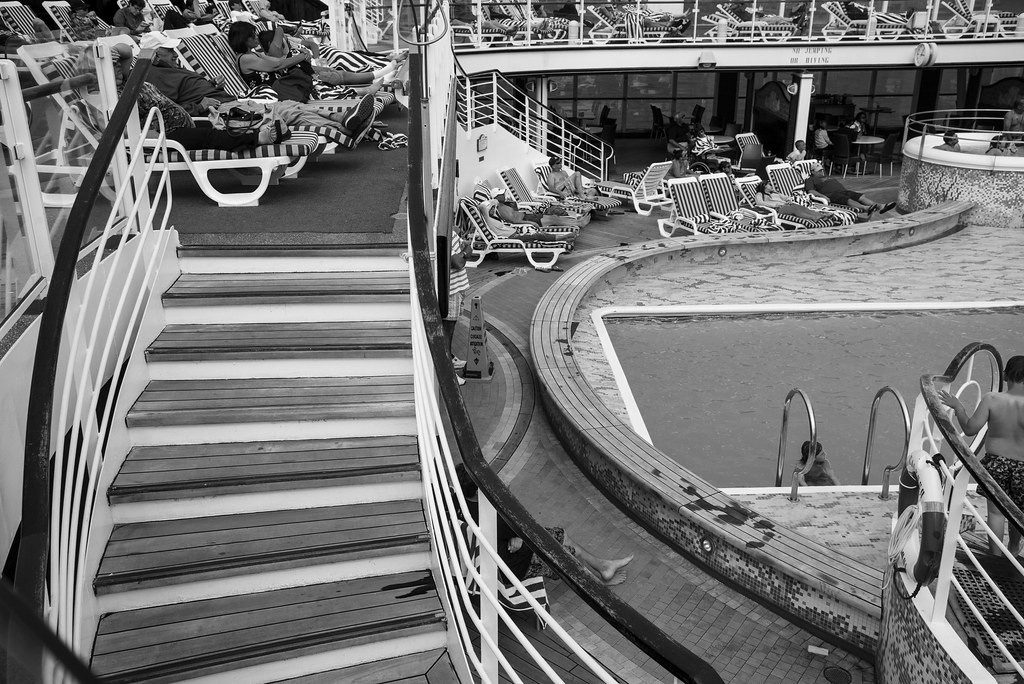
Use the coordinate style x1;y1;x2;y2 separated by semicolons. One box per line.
549;156;562;166
490;188;507;199
140;31;182;49
70;0;90;11
811;163;824;172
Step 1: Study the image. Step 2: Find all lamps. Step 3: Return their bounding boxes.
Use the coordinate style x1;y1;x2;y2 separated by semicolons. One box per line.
787;83;798;95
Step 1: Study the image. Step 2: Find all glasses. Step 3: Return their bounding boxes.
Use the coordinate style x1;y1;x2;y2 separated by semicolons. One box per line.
556;160;561;164
133;5;142;11
679;115;685;119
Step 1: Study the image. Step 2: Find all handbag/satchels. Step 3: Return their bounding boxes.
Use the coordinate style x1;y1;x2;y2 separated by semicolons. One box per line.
219;106;263;137
272;67;313;104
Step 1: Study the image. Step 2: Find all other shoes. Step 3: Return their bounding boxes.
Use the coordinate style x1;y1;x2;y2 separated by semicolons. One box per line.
351;107;376;145
879;201;896;214
452;353;467;369
342;93;374;130
456;373;466;386
867;203;877;216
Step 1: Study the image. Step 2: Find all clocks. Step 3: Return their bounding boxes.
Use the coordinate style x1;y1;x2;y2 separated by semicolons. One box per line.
914;42;939;67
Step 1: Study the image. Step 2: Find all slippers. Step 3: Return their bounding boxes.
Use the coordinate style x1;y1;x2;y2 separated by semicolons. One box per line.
265;119;282;144
372;121;389;127
624;209;636;212
608;211;624;214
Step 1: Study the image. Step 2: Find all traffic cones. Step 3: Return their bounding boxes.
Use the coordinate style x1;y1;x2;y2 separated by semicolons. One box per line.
462;294;497;382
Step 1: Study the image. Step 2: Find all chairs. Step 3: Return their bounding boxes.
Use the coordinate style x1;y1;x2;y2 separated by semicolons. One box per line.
792;159;873;222
97;34;356;181
0;0;1020;45
828;133;860;177
495;167;591;229
472;178;580;243
549;105;774;173
698;173;783;231
454;198;573;268
862;133;899;177
736;175;839;229
533;164;620;219
766;162;857;226
599;158;673;216
165;29;383;138
451;517;549;629
19;41;318;206
658;176;749;234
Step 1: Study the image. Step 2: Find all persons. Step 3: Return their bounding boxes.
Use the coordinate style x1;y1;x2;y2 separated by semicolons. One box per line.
76;43;282;149
785;140;807;165
140;32;376;145
450;0;886;35
838;116;857;171
815;121;836;170
985;100;1024;155
846;112;872;154
805;163;897;216
228;21;384;100
662;123;730;198
755;181;834;223
479;188;574;242
796;441;842;486
259;26;398;85
933;131;960;152
548;156;599;201
71;0;327;68
936;355;1024;559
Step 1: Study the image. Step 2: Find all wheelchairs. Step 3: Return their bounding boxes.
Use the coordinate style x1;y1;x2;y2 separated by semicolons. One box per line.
688;143;724;174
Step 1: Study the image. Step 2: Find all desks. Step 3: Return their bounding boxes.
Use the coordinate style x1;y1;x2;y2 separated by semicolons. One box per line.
662;110;694;123
571;127;602;161
859;107;894;136
689;125;722;132
699;136;733;144
566;114;595;126
851;136;885;161
